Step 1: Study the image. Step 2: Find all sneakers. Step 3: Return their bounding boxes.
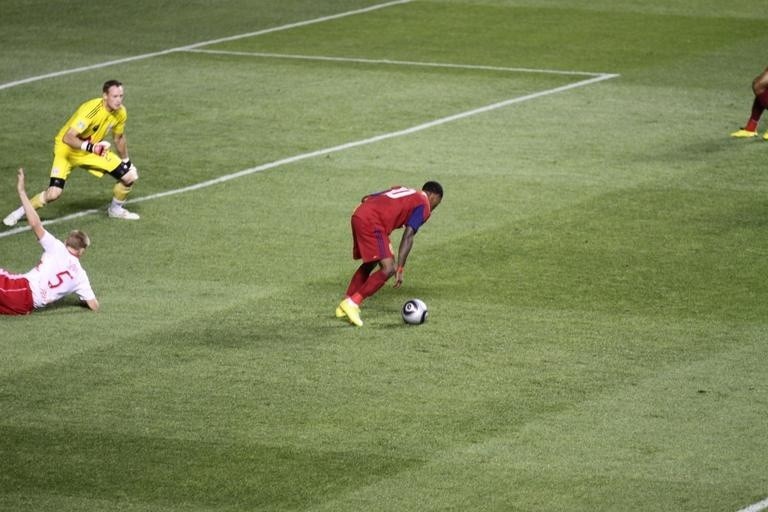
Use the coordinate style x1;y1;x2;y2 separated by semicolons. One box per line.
763;129;768;140
3;209;23;227
336;306;361;318
107;206;139;221
339;298;363;327
731;128;758;137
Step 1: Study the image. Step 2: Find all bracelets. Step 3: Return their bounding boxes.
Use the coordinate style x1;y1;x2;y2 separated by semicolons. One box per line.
396;266;405;272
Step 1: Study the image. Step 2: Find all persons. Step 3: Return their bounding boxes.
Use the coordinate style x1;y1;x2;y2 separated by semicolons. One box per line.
2;80;140;226
730;68;768;140
0;166;99;316
336;182;443;327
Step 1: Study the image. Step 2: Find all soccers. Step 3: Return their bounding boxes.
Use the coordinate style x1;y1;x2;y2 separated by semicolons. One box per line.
402;298;427;324
